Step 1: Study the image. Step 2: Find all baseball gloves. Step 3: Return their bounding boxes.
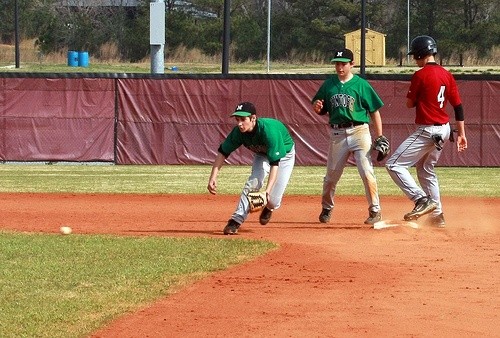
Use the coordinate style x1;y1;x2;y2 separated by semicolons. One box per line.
246;191;268;214
373;135;390;161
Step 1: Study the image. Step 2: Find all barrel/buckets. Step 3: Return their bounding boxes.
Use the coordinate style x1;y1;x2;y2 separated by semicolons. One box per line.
78;51;89;67
68;50;78;67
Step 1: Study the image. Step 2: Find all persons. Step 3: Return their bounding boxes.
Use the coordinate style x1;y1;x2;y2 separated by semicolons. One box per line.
208;102;295;235
386;36;468;228
311;49;390;225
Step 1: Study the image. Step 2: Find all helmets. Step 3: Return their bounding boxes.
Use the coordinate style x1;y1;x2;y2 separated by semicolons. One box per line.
406;36;438;60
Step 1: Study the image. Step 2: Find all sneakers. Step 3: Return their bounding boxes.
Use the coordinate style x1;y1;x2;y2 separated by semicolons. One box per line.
428;212;446;230
260;204;272;225
403;194;439;220
364;210;381;224
319;208;333;223
224;219;241;234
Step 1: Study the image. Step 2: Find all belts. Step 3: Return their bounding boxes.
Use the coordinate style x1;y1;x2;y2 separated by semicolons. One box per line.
434;123;442;126
330;121;364;129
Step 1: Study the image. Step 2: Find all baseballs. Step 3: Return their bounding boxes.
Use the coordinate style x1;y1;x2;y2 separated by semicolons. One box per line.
60;226;73;235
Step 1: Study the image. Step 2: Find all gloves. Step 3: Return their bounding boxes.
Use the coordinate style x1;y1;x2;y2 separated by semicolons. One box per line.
449;128;455;143
432;135;445;151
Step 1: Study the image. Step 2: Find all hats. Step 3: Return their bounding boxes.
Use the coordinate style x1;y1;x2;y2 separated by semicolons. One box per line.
229;102;256;118
331;48;353;63
